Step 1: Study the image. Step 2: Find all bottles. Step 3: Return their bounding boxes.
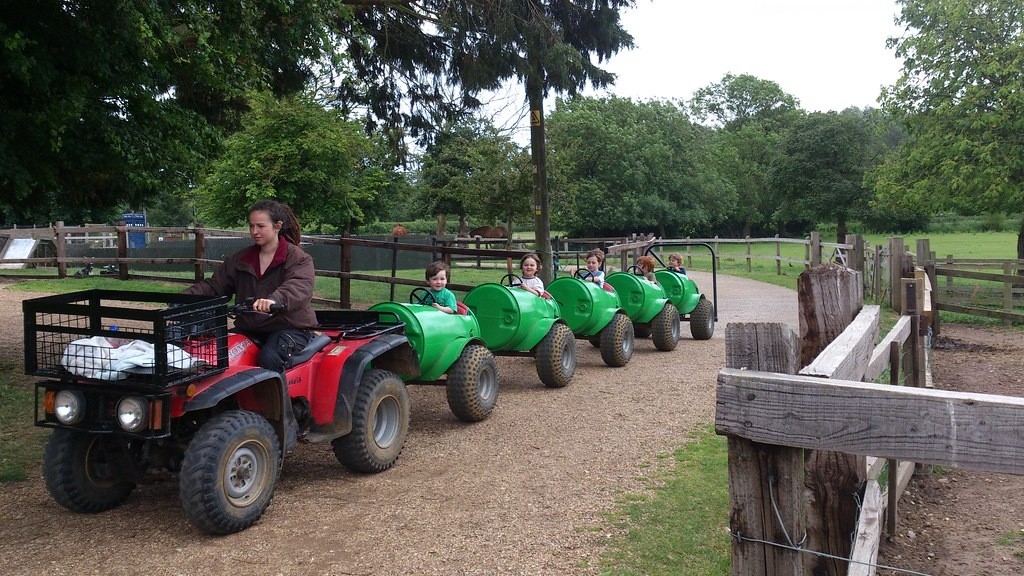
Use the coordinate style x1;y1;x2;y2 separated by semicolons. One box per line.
107;325;126;346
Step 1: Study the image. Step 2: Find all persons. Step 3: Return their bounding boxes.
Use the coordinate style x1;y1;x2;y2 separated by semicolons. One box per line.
512;254;544;298
417;262;457;314
570;248;605;288
633;256;658;285
178;200;318;451
666;254;686;275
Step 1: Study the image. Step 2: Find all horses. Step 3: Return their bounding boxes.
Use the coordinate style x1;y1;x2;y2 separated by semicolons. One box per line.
469;225;512;250
390;221;408;238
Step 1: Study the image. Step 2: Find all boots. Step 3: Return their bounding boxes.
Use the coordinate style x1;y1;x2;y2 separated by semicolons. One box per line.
279;365;300;450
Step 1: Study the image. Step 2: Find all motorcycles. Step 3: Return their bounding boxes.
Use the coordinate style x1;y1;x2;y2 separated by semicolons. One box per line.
100;264;120;275
21;243;421;537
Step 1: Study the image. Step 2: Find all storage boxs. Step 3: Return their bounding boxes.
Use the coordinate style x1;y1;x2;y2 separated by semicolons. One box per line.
22;287;230;389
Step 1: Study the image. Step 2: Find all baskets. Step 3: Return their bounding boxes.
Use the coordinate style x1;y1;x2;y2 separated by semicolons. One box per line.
21;289;230;390
